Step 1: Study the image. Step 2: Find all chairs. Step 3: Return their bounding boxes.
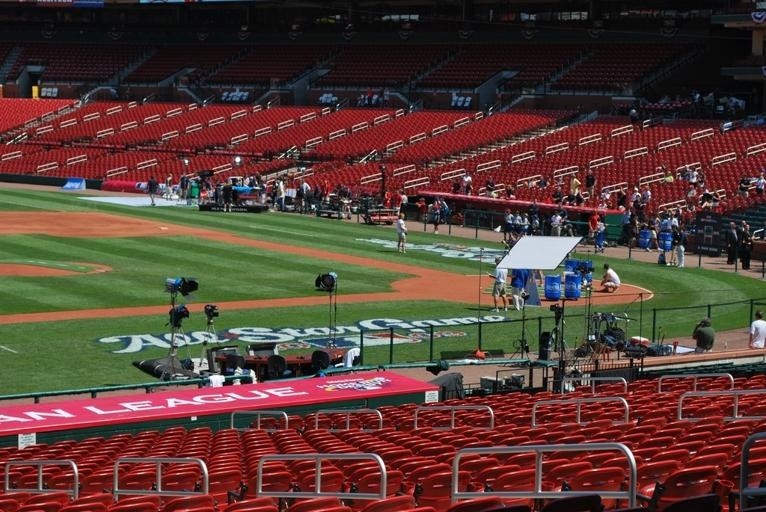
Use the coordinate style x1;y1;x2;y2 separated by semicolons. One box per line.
2;41;765;249
0;362;766;512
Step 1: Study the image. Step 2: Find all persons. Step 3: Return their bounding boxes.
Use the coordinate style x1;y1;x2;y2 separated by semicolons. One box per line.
219;87;748;123
487;256;509;313
533;269;544;288
600;263;622;293
692;317;717;353
507;269;529;310
748;309;766;349
143;162;766;270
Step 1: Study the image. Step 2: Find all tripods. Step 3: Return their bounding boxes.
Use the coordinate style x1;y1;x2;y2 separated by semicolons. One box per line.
503;299;532;367
199;325;220;367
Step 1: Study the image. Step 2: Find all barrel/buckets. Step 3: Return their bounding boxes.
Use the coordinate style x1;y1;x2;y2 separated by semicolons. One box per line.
544;275;561;300
564;273;581;299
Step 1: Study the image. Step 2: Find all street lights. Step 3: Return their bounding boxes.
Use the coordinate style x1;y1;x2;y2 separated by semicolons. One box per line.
184;159;189;174
235;156;241;176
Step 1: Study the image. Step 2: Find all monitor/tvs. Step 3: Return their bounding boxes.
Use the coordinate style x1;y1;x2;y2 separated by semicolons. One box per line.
211;347;238;372
249;345;279;358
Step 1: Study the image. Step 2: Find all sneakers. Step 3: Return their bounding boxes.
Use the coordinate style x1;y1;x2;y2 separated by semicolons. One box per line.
491;307;507;312
601;287;619;293
